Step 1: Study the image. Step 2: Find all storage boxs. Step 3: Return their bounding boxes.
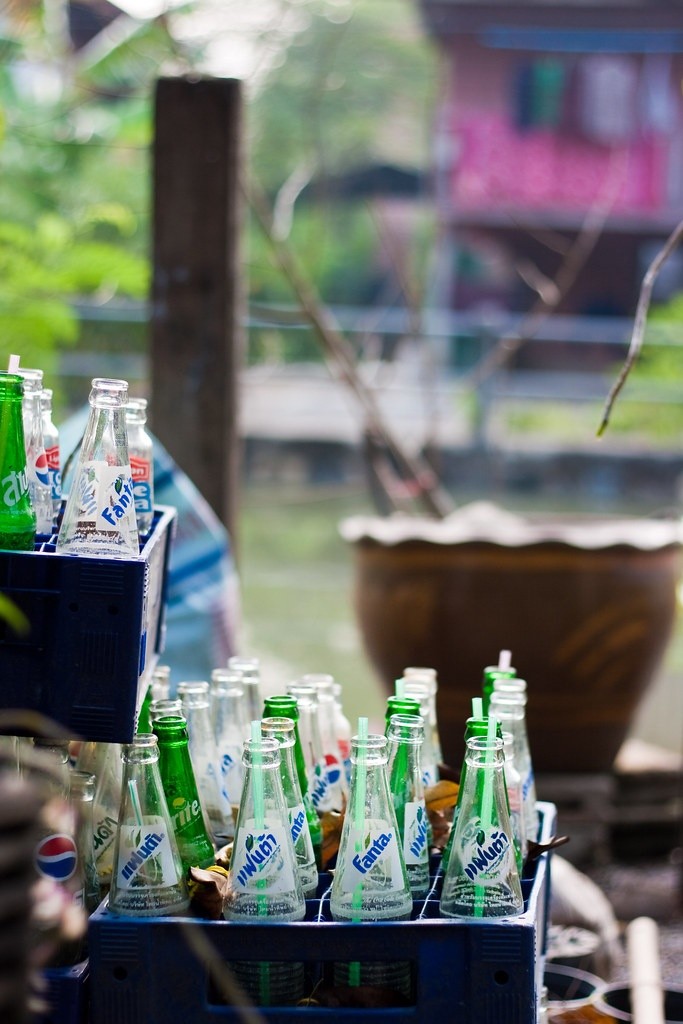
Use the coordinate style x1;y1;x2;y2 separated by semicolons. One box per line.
0;505;555;1024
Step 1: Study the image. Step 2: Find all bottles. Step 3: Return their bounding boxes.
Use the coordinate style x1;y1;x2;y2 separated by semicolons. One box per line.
0;651;537;923
0;366;157;559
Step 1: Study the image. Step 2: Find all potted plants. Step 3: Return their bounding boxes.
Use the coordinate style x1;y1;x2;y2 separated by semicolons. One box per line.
72;0;683;771
